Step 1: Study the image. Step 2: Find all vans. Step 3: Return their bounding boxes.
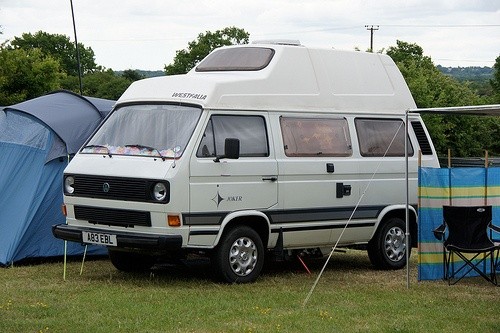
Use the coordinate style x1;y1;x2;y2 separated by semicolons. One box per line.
51;37;442;284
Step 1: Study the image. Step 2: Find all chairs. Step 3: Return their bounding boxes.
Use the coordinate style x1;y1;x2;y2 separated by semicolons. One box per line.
432;205;500;286
